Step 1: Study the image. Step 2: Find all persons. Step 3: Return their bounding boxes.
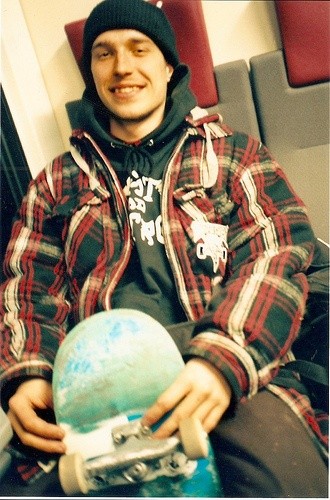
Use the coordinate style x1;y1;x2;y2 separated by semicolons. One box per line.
0;0;329;498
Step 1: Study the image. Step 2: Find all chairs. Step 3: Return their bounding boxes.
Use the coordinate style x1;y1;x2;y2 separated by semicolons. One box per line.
64;0;330;247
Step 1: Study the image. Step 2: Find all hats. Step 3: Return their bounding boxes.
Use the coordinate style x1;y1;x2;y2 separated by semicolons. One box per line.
81;0;179;95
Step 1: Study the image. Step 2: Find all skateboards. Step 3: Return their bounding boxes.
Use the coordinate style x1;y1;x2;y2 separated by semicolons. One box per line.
52;308;224;498
